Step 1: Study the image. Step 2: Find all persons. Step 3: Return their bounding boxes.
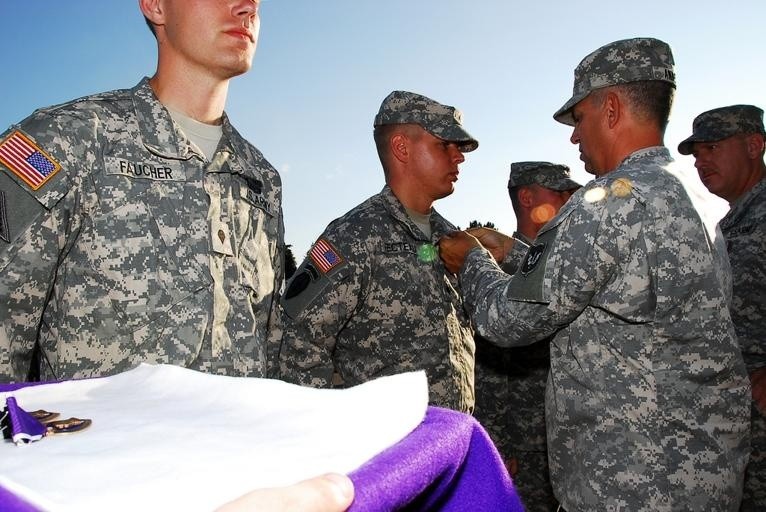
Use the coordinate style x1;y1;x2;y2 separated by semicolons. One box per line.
211;470;357;512
1;1;286;397
678;104;766;511
277;89;478;416
437;37;753;511
474;161;584;511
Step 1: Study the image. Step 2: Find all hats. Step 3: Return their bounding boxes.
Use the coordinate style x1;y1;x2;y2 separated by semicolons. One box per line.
373;90;478;153
507;162;585;193
679;105;764;155
553;38;676;125
